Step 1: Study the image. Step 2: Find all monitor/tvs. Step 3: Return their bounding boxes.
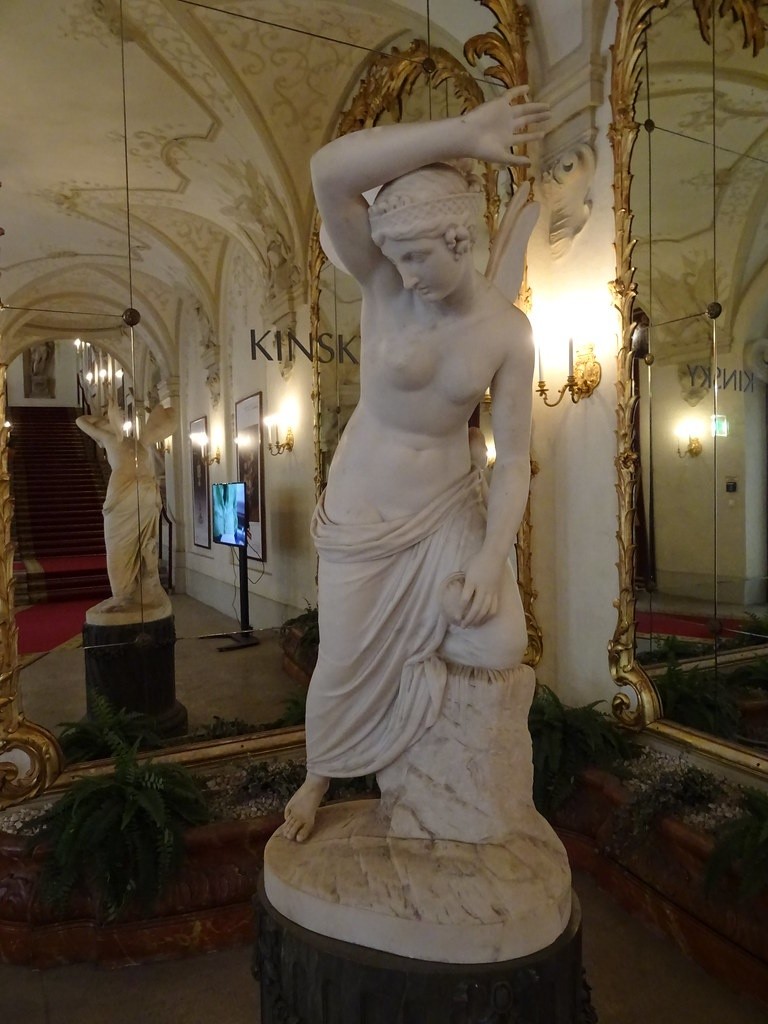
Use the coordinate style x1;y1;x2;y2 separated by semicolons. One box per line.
212;482;248;547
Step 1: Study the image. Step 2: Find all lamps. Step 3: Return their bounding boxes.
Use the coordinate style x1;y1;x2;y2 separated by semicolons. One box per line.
265;419;294;457
535;335;602;407
678;436;704;460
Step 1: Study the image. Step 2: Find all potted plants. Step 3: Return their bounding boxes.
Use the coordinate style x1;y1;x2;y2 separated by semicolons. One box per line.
278;599;319;685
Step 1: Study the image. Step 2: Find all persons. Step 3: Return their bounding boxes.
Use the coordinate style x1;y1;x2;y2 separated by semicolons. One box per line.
77;402;178;613
284;84;535;846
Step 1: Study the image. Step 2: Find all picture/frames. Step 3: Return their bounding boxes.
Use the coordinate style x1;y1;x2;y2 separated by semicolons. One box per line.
189;416;212;549
234;391;267;564
23;340;56;400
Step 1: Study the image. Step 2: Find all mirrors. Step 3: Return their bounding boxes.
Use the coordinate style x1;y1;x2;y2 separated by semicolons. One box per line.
0;0;543;810
609;0;768;781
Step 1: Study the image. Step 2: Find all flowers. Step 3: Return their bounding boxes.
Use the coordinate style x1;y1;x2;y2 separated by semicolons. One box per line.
593;746;752;865
